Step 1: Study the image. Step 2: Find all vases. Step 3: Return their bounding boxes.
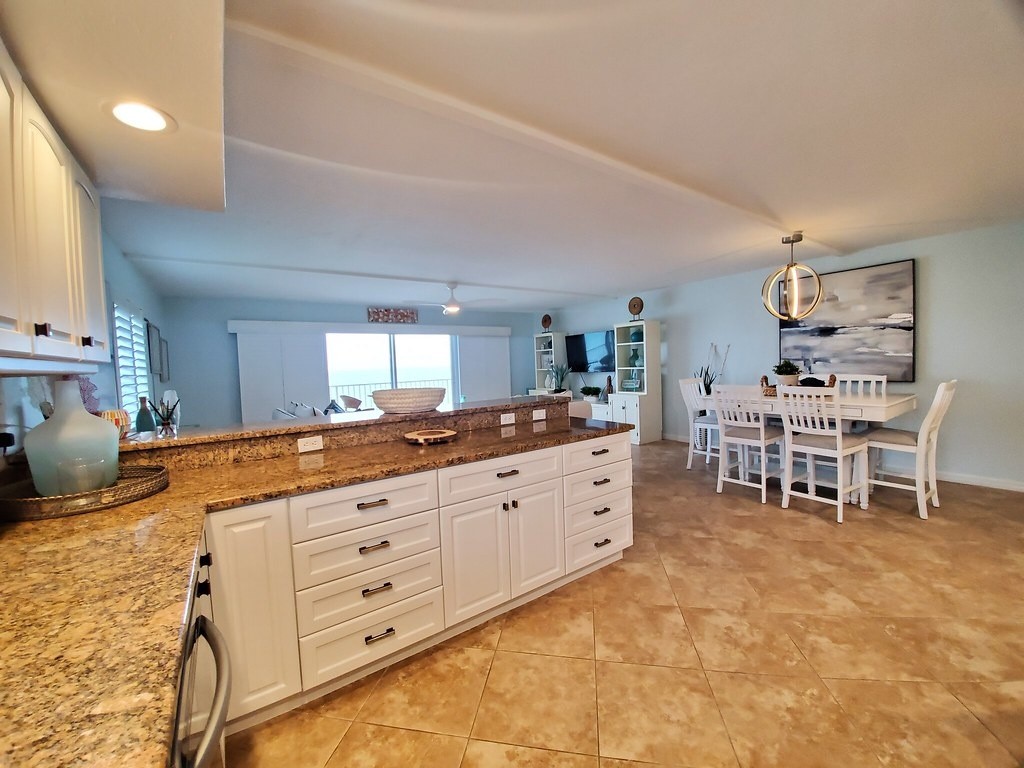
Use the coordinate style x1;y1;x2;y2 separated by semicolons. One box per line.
136;397;155;432
23;380;119;497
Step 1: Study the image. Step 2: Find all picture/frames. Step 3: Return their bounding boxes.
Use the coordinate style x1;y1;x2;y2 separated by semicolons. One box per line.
779;258;916;382
147;323;174;382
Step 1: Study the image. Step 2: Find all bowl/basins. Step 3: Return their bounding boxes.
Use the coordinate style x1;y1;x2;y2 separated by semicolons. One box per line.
371;387;446;415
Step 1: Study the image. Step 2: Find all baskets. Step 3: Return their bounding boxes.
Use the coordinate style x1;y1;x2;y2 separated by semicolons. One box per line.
372;388;446;413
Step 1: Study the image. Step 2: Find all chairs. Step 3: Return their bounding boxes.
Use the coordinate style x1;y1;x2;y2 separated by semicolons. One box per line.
679;373;958;523
340;395;364;411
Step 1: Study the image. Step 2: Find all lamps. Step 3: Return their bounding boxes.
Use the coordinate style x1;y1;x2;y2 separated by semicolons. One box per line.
761;233;822;321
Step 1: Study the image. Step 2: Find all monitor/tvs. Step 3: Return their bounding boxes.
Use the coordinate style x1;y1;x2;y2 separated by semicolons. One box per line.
565;330;616;372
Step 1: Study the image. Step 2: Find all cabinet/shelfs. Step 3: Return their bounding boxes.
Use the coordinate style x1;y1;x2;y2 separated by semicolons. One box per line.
528;321;662;445
188;430;634;748
1;41;112;373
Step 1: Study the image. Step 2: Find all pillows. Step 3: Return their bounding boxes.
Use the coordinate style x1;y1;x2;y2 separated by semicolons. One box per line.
288;401;326;418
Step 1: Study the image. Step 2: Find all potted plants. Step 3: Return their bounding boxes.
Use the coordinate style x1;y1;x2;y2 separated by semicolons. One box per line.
772;361;802;386
547;364;574;393
692;366;718;449
580;386;601;401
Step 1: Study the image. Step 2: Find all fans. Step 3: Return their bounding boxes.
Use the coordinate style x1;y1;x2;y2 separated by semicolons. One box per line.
403;283;507;315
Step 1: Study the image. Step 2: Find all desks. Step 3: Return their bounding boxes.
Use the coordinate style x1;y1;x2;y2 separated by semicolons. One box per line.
697;388;917;503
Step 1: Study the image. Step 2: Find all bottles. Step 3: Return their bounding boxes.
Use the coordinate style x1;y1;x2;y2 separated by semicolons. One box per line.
629;348;639;367
23;379;120;496
545;373;551;388
135;396;157;434
635;354;644;367
